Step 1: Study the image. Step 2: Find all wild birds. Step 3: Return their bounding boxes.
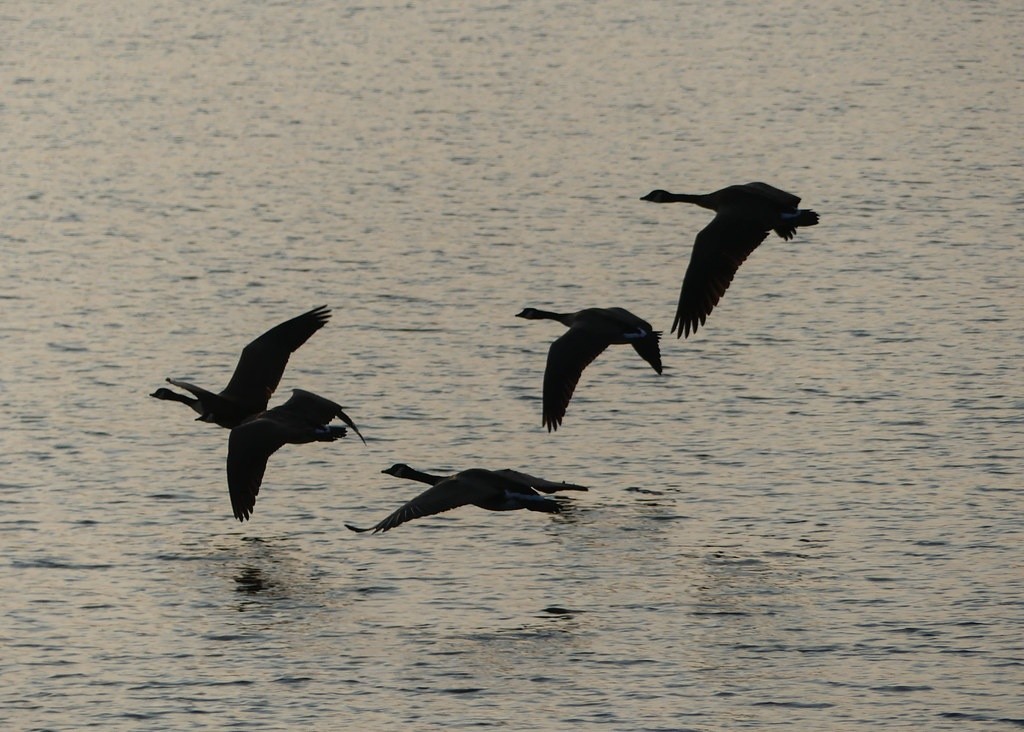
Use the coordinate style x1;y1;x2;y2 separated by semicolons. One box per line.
344;462;589;535
149;304;368;524
640;181;820;340
515;307;664;433
